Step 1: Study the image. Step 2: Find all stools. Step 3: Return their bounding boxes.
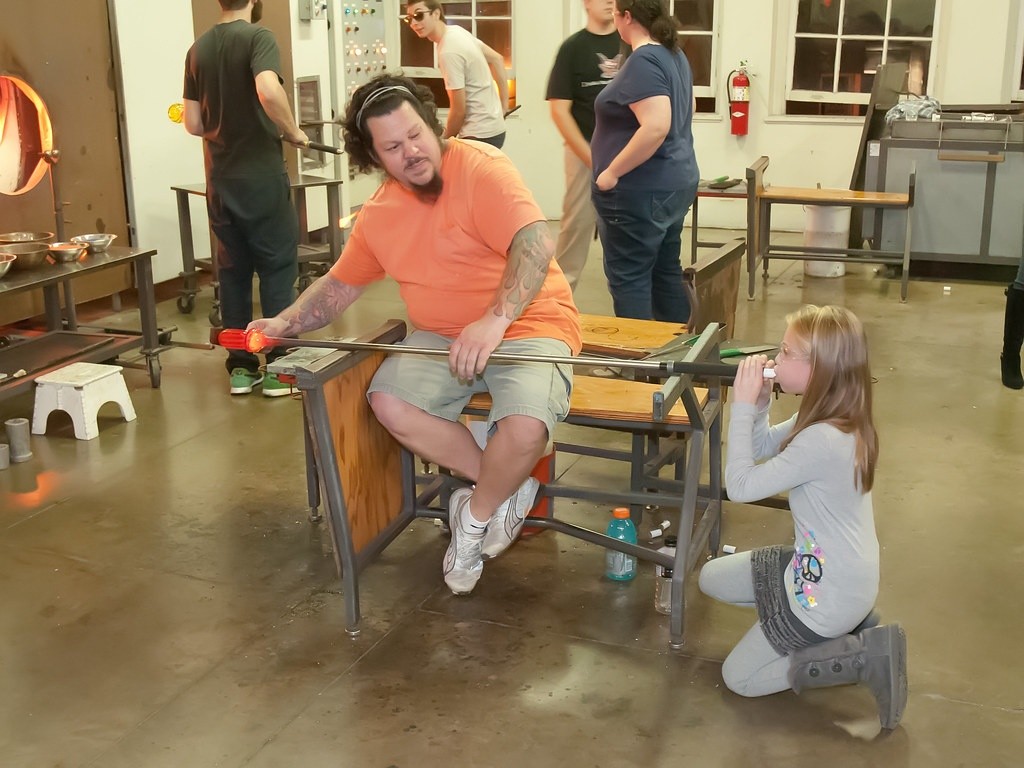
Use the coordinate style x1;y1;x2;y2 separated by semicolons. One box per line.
30;361;138;441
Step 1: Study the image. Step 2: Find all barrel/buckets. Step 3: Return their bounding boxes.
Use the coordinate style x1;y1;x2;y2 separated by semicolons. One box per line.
802;188;852;278
517;444;557;536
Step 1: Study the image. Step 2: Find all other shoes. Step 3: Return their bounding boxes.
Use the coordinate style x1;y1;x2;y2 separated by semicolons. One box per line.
589;366;622;377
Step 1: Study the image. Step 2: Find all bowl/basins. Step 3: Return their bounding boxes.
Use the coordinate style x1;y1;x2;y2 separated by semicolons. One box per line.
0;243;50;270
0;252;18;278
49;241;90;262
70;233;119;253
0;230;55;245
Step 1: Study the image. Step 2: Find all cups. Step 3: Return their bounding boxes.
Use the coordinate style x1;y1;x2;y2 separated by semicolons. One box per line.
904;100;918;122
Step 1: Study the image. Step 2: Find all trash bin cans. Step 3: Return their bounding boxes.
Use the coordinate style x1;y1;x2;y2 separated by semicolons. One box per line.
801;200;853;279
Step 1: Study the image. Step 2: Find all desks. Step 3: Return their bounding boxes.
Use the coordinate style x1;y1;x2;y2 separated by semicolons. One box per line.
0;246;178;402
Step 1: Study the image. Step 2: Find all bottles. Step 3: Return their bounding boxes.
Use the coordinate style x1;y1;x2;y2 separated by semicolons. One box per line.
604;507;638;582
167;103;184;124
655;535;688;616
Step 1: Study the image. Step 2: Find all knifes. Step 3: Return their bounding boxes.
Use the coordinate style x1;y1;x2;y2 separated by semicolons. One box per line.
720;344;778;358
640;334;702;360
698;175;729;187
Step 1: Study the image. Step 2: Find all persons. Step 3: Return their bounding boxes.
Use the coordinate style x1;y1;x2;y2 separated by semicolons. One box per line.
1000;261;1024;390
401;0;511;148
546;0;632;295
698;305;907;729
245;75;582;596
591;0;700;322
182;0;309;397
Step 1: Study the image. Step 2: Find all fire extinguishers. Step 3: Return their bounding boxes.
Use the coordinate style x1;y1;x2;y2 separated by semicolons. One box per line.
726;60;757;136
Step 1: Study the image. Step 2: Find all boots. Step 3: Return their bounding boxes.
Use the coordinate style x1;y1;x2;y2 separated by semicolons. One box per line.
787;608;907;730
1000;286;1024;389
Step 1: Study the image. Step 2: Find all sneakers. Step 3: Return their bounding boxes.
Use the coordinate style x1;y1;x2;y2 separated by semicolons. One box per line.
229;368;264;395
263;372;300;398
442;477;540;596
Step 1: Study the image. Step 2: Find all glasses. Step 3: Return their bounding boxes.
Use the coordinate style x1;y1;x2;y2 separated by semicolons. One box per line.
404;11;431;25
778;342;811;362
